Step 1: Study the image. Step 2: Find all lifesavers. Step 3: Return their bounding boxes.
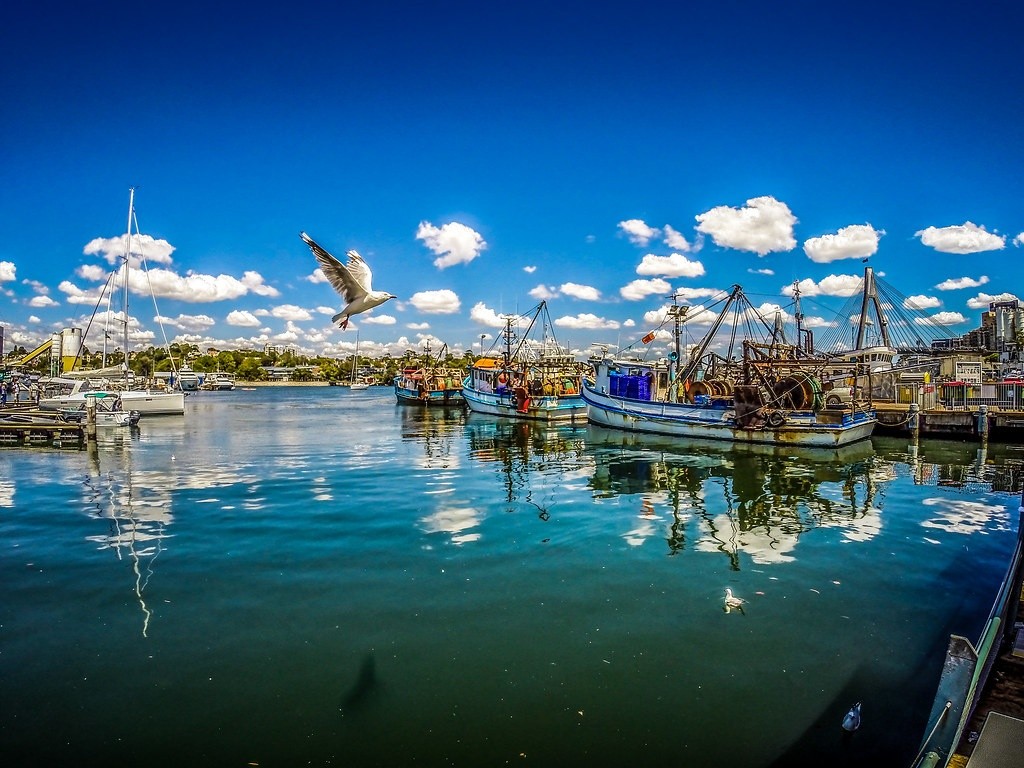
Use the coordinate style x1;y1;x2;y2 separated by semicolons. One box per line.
511;395;518;405
756;410;785;428
101;383;113;391
825;394;841;404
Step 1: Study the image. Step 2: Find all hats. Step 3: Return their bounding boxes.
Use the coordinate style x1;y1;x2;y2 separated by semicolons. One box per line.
15;381;19;383
2;382;6;384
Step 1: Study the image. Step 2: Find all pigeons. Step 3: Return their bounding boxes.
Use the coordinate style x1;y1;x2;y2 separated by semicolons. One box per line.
298;230;398;330
842;701;861;731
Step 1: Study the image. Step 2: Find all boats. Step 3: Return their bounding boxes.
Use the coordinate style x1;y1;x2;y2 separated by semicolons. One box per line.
0;184;236;419
330;258;1023;573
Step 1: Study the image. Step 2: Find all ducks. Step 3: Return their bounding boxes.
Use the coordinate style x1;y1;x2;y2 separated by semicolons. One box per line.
724;605;746;616
724;588;744;606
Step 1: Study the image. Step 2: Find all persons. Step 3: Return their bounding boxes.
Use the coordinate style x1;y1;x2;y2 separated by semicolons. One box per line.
112;396;123;411
1;381;20;407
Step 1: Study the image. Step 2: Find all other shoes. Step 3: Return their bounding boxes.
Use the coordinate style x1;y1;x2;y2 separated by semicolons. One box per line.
4;405;7;407
13;404;17;406
2;403;3;406
18;405;20;406
